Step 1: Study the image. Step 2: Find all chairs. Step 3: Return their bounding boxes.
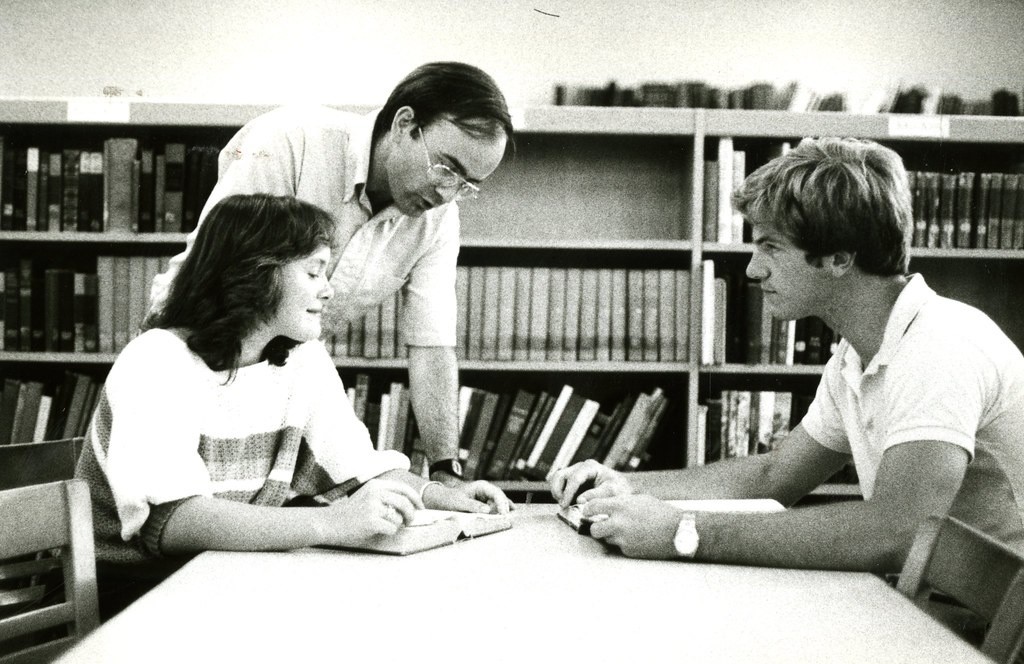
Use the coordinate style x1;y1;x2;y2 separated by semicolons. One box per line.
892;514;1024;664
0;477;97;664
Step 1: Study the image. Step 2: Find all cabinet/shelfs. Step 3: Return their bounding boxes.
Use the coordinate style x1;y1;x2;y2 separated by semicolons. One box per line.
0;95;1024;644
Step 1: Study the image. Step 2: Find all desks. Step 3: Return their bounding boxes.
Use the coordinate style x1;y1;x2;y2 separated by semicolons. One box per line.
46;499;998;664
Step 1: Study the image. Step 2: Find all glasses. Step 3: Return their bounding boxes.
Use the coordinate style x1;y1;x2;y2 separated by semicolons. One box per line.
417;124;480;202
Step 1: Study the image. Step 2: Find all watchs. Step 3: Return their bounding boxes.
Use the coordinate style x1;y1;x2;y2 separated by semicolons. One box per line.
673;511;699;558
429;458;463;480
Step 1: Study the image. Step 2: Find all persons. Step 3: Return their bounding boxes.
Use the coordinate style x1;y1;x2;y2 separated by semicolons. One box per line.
550;135;1024;644
42;193;510;634
150;62;517;510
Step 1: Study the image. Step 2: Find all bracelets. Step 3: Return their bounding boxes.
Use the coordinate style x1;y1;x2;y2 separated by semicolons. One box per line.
420;481;444;499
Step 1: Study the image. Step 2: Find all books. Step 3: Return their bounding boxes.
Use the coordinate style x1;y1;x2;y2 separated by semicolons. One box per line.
311;500;511;556
697;138;1023;468
0;145;222;445
557;499;787;535
322;269;688;502
